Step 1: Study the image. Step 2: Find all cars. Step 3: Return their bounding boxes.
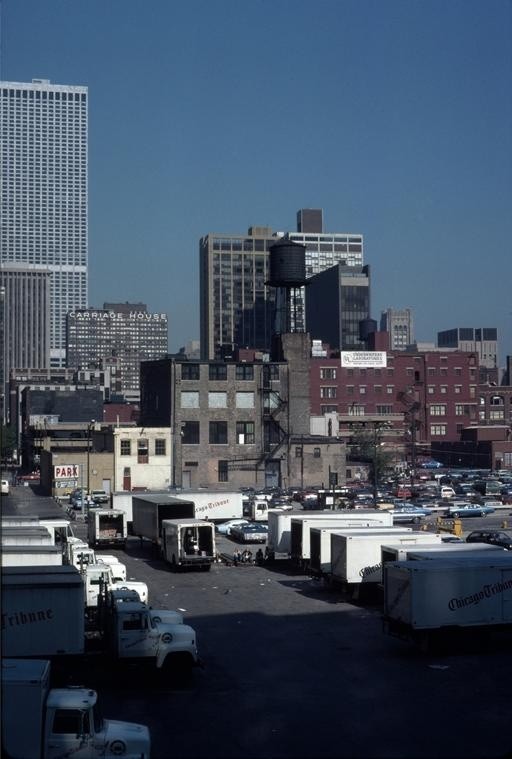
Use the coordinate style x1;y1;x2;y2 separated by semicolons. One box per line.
216;519;270;542
69;486;109;509
0;479;10;496
239;469;512;524
421;459;444;469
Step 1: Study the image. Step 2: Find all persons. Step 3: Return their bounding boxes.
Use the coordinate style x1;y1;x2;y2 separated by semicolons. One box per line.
232;546;275;566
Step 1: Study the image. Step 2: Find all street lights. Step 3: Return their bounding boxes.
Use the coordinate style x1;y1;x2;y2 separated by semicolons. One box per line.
87;418;96;517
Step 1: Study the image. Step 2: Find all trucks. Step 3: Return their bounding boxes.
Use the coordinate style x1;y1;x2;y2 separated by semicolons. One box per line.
1;658;153;759
109;491;268;523
0;573;199;691
0;514;184;625
268;510;512;647
84;494;215;571
